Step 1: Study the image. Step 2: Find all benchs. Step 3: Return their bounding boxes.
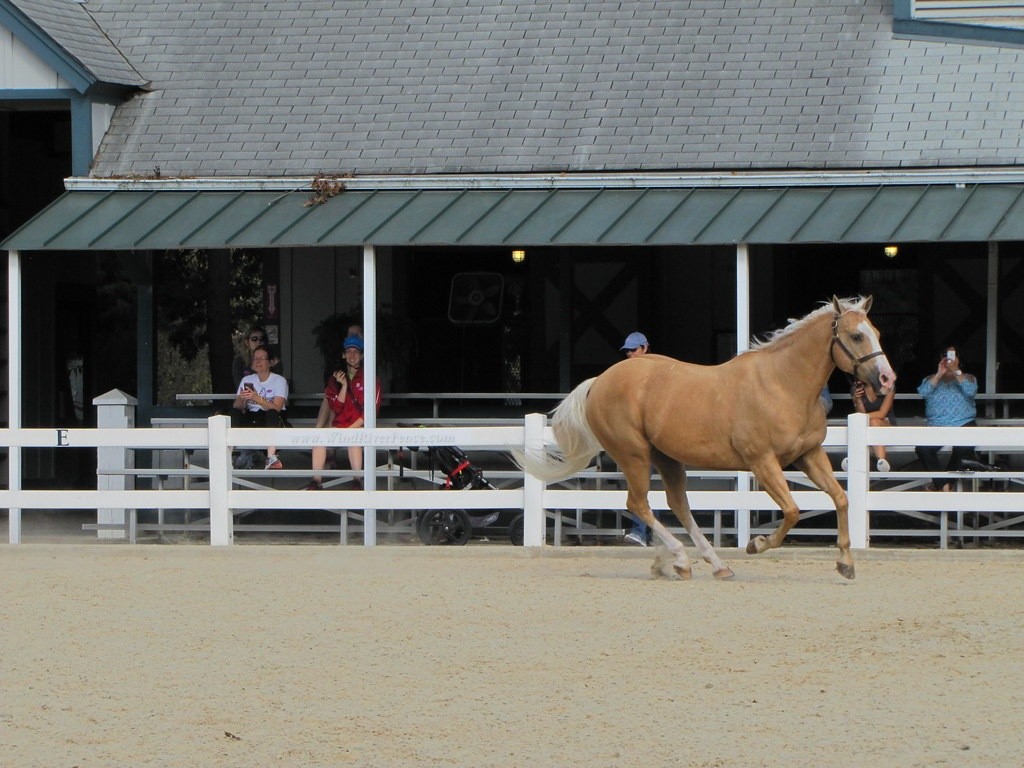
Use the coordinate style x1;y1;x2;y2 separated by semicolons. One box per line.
139;391;1024;534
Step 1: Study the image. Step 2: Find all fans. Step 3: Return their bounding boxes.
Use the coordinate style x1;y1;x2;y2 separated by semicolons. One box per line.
448;270;504;324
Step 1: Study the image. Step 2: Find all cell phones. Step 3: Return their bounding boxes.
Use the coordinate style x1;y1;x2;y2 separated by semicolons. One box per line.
244;382;254;392
857;382;862;388
947;351;956;362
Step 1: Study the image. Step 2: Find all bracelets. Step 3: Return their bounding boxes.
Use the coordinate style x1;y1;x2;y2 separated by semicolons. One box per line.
936;375;941;380
260;397;262;405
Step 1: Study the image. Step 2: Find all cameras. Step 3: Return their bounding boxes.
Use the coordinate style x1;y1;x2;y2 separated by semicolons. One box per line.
336;358;347;375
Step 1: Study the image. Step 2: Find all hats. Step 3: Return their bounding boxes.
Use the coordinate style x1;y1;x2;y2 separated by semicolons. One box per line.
619;332;650;351
344;335;364;352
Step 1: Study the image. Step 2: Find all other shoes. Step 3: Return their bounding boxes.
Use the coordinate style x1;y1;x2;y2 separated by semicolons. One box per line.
841;457;848;471
352;478;362;490
877;459;890;472
306;480;322;490
925;482;936;491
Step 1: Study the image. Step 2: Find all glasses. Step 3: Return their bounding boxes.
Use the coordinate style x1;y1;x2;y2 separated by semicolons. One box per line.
249;336;265;342
626;347;642;352
253;356;269;360
942;351;947;357
344;349;361;355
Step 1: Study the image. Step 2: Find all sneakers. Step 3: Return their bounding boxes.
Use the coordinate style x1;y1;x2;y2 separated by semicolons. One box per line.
265;455;282;469
236;452;248;466
624;532;647;547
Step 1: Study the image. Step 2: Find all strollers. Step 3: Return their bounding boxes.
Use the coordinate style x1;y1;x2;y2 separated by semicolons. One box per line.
395;420;525;546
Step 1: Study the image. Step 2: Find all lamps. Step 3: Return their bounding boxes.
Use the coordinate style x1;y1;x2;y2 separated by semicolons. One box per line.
512;248;526;263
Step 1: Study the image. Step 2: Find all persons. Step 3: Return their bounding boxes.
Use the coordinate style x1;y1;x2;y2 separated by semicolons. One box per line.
620;331;659;547
819;381;896;473
230;322;383;491
915;345;978;492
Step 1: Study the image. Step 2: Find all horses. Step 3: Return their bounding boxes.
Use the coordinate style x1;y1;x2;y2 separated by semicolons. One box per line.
503;293;897;584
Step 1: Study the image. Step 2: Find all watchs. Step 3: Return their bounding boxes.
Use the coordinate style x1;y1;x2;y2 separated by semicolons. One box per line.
954;369;962;376
